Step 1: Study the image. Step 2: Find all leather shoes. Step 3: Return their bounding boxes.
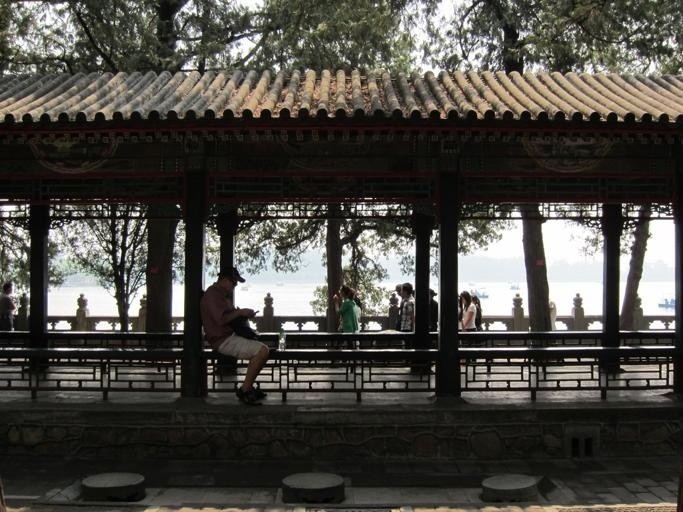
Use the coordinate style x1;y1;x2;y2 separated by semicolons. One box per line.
235;386;267;406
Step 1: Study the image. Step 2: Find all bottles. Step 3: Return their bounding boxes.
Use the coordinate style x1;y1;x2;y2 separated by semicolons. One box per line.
278;329;287;353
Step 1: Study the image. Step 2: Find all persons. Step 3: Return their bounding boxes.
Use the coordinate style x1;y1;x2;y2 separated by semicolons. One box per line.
395;282;482;363
0;282;16;331
333;284;358;348
200;267;270;405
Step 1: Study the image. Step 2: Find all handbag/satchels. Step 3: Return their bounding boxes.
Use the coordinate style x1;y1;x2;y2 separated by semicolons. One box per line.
227;308;257;339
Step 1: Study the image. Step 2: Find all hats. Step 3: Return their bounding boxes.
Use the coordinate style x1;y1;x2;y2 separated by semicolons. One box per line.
217;268;246;283
